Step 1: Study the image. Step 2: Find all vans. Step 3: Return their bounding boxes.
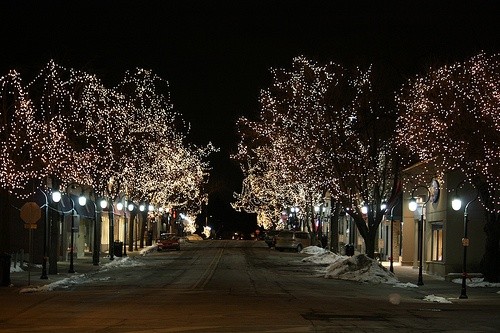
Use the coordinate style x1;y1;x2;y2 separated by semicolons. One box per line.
276;230;322;253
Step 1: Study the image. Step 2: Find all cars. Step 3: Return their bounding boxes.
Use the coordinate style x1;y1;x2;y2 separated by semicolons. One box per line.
265;230;280;248
157;232;181;251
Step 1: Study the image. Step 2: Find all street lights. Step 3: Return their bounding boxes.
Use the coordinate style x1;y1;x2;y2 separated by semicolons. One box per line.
380;195;399;275
289;198;323;254
51;188;173;274
407;184;431;285
451;179;480;299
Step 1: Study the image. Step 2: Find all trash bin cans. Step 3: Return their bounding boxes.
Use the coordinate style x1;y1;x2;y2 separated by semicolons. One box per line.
114;239;123;257
